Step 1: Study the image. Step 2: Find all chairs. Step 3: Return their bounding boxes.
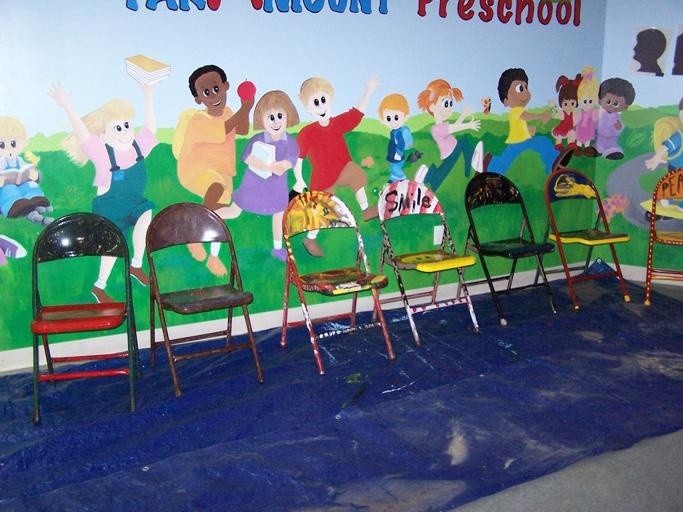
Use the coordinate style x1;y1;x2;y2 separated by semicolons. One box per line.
372;180;480;347
280;191;396;375
456;172;557;327
644;168;683;306
31;212;143;425
534;168;631;311
145;203;264;398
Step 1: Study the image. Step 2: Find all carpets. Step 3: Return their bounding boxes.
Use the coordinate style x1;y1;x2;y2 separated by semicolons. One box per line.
1;260;683;512
